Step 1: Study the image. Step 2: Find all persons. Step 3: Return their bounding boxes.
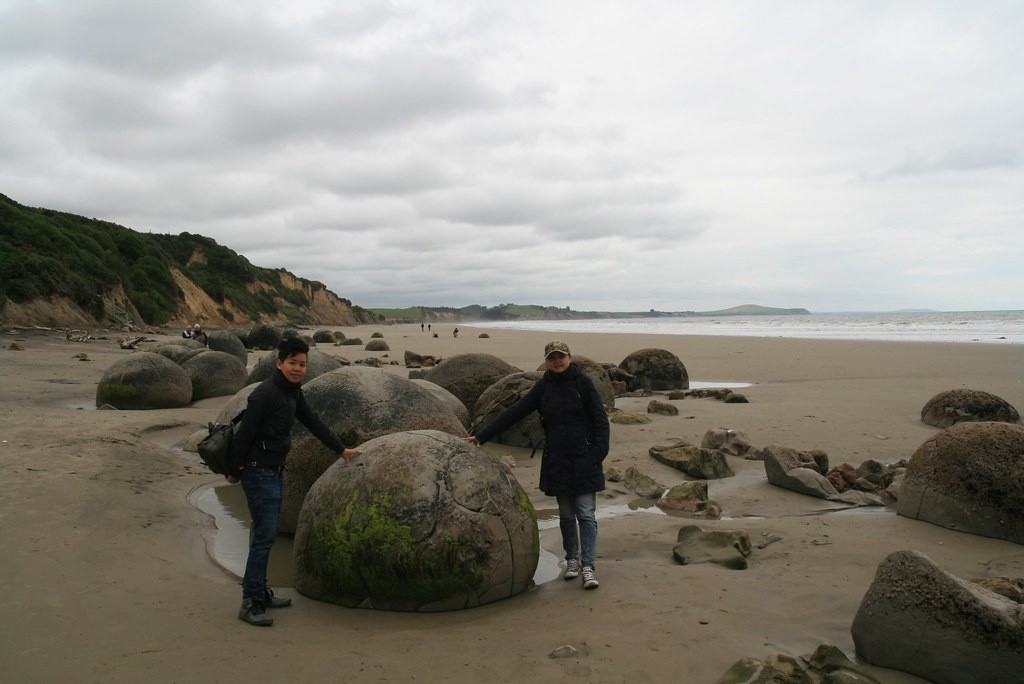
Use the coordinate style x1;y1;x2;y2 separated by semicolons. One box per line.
462;340;610;588
182;324;208;346
225;338;364;626
453;328;459;337
421;323;424;331
428;324;431;331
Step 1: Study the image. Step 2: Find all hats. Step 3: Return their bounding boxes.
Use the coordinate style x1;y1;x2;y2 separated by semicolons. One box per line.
544;340;570;359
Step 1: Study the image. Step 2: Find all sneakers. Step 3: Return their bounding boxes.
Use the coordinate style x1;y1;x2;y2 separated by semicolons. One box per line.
582;566;599;589
564;557;581;577
263;585;291;608
239;594;274;626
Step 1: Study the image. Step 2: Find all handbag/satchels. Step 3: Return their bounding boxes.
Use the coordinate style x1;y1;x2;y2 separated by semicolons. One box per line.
198;426;234;474
